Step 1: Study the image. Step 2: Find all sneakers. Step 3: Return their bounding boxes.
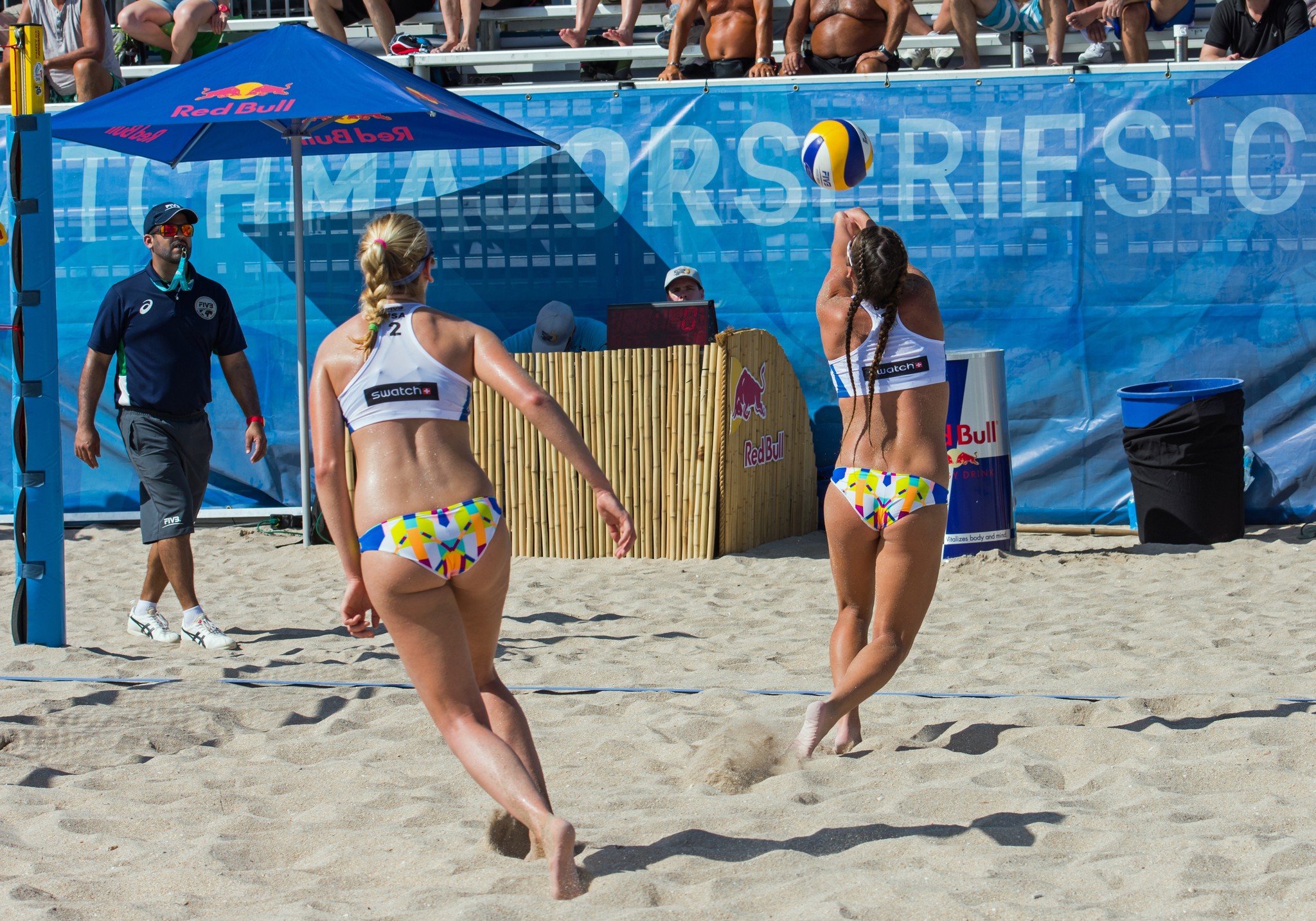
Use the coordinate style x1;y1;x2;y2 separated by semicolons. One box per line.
653;4;705;50
928;31;956;69
181;614;238;651
1023;44;1036;65
900;48;930;70
1078;42;1113;64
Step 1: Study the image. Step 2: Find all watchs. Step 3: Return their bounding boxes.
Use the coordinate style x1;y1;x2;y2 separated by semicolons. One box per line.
878;44;892;60
754;57;771;65
218;4;230;12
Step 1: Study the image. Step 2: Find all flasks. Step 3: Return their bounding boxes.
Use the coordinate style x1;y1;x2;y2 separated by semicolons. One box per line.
1173;24;1188;63
1009;31;1024;67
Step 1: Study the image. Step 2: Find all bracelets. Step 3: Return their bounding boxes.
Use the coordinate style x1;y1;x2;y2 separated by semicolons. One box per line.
245;415;267;427
667;62;681;69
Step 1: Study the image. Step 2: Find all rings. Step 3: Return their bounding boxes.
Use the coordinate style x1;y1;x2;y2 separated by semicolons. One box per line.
766;72;771;74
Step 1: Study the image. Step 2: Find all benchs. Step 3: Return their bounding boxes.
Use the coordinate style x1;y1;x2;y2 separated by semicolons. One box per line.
111;0;1232;78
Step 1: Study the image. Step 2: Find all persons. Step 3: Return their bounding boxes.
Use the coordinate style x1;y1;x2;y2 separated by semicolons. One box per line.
73;200;268;652
503;300;607;354
790;206;953;760
308;0;435;59
430;0;518;53
558;0;642;49
664;264;733;333
0;0;232;105
778;0;1316;76
307;208;635;901
655;0;776;81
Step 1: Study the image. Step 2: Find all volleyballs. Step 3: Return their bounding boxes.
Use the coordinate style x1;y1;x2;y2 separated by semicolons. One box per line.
801;119;873;191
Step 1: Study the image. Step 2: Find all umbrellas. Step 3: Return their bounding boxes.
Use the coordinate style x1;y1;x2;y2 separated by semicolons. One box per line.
47;21;563;543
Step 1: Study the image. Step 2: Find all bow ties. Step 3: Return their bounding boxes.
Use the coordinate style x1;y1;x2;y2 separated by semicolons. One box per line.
127;606;180;643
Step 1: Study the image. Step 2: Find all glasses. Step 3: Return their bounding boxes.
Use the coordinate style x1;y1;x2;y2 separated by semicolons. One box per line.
149;224;194;237
847;233;858;276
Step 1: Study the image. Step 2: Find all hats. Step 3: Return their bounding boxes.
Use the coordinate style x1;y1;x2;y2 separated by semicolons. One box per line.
144;201;199;235
664;266;703;293
531;300;575;359
